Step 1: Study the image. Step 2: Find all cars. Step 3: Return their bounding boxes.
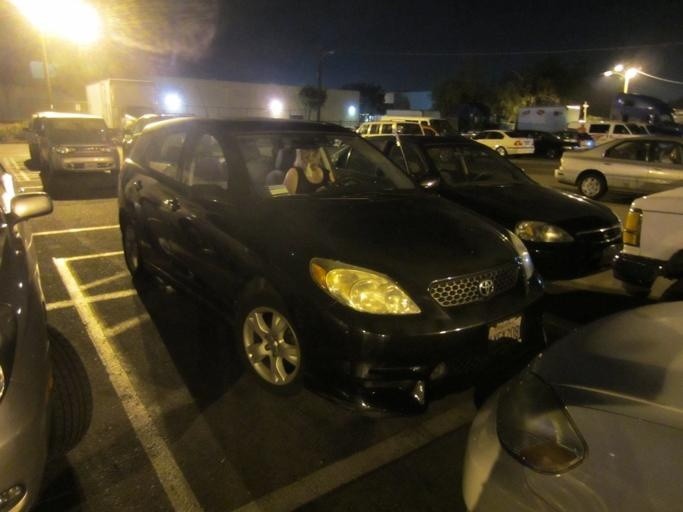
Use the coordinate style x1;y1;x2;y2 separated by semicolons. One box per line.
122;113;202;163
333;134;627;282
554;134;682;199
516;96;682;143
0;166;95;512
615;188;683;292
469;129;537;159
23;111;120;183
120;113;546;406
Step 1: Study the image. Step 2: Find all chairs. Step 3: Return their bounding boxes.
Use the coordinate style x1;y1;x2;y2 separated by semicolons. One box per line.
443;148;471;177
242;143;296;186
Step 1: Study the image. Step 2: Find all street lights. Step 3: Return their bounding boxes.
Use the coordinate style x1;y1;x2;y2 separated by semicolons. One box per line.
602;58;642;93
314;49;336;92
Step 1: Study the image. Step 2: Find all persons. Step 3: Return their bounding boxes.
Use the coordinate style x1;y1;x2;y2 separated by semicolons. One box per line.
281;145;339;197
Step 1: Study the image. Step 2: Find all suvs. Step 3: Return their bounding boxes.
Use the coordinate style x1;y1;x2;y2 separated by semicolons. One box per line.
351;121;425;138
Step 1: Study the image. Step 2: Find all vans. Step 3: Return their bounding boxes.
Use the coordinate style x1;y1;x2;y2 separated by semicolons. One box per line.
381;115;459;136
